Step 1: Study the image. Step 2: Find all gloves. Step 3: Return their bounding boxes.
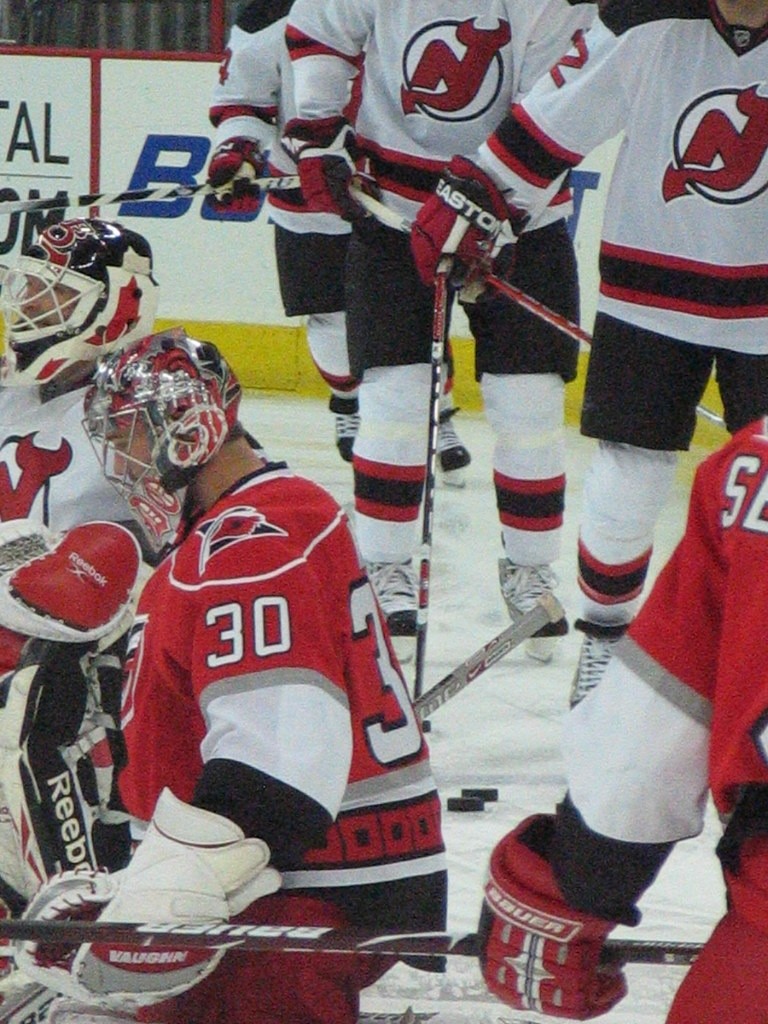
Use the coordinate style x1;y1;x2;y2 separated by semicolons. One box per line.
66;785;281;1003
284;114;382;220
202;133;265;210
478;810;630;1019
406;151;533;291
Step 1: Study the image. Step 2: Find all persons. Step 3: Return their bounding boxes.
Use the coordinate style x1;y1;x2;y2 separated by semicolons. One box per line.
196;0;768;707
0;211;452;1024
479;423;768;1024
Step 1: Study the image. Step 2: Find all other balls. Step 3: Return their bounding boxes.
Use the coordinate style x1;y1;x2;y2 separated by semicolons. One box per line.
447;787;502;813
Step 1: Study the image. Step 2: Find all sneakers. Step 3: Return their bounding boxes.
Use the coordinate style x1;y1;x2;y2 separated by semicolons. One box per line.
363;559;419;667
436;403;472;489
327;390;361;464
494;554;569;662
570;614;633;710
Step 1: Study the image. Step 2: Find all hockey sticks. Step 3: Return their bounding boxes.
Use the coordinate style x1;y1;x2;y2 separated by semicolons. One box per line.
402;253;450;729
345;180;727;445
1;916;703;968
1;173;309;218
411;590;567;729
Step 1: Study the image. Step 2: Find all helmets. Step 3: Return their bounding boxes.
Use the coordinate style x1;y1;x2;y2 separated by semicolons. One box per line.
8;220;161;388
82;325;244;493
